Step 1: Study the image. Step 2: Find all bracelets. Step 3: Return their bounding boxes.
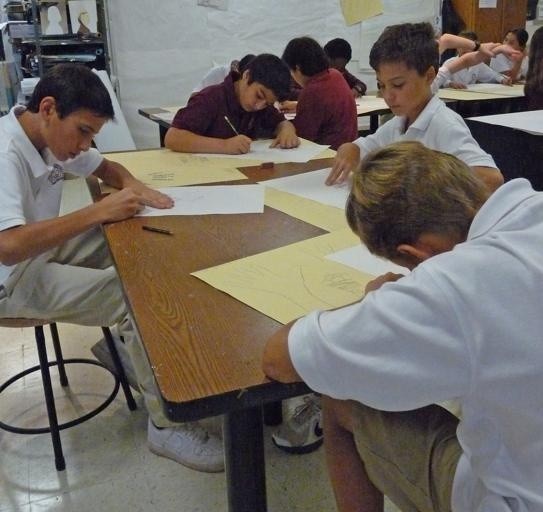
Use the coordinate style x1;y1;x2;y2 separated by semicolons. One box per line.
353;85;362;93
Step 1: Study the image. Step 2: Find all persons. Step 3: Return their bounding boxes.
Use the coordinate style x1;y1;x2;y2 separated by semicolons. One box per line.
184;54;257;108
270;21;503;455
280;36;359;151
261;139;543;512
164;53;301;155
0;60;226;474
278;38;366;101
430;27;530;94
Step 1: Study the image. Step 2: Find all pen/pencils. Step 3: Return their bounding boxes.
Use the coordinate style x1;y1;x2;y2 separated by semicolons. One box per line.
224;116;239;136
142;224;173;235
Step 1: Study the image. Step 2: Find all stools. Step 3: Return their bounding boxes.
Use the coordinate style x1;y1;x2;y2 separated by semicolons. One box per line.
0;313;136;470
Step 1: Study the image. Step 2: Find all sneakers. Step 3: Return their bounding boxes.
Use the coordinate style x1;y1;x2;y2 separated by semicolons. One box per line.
146;415;225;473
271;393;325;455
90;323;142;394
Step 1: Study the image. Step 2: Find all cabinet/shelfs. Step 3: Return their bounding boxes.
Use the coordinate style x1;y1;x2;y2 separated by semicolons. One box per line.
453;0;527;44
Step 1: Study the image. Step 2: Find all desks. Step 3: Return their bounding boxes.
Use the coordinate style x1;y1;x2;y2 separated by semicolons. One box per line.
85;81;542;511
